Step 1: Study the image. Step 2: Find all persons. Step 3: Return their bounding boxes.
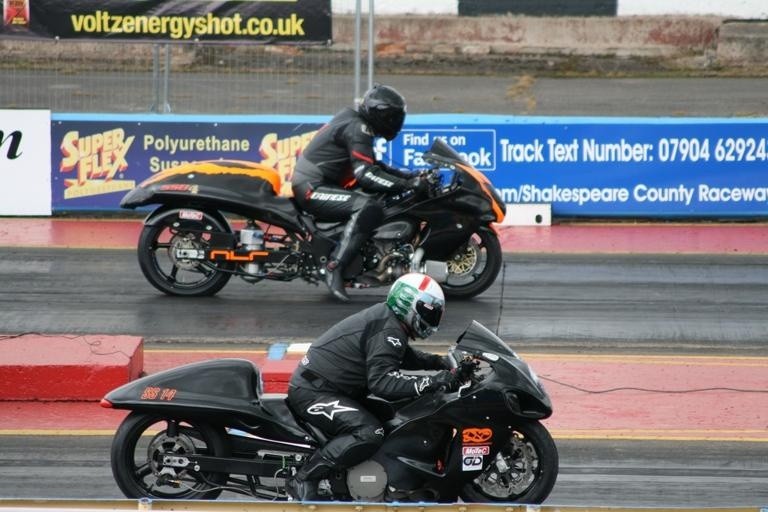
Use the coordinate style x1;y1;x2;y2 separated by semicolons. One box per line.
291;83;430;304
282;272;458;503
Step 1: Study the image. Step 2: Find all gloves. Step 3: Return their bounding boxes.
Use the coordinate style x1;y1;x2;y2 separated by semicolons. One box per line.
406;168;432;177
423;355;451;371
411;172;442;196
416;370;464;396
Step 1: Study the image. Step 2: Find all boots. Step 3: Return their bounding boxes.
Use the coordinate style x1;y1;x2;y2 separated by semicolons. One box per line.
284;448;338;501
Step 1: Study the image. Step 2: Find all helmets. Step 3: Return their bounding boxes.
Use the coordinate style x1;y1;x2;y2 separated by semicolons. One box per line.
386;272;446;341
359;82;406;141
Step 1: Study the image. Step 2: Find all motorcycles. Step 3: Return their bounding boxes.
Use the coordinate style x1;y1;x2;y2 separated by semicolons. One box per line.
99;320;558;504
119;137;506;299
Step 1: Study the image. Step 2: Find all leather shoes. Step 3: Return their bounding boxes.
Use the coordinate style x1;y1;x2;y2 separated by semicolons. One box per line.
316;258;350;303
